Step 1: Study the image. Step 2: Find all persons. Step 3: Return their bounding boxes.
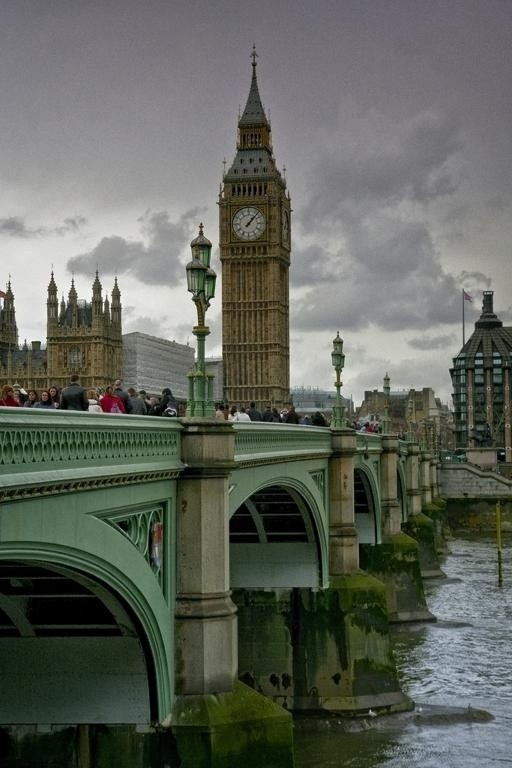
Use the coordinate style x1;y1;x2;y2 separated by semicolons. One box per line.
346;420;382;435
215;401;327;427
0;375;180;418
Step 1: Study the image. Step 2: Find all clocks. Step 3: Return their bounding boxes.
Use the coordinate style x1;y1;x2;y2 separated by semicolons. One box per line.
232;208;266;240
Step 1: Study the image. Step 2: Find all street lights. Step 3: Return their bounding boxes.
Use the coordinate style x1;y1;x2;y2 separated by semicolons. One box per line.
330;330;346;428
381;372;392;435
418;416;440;450
406;399;415;440
185;222;218;419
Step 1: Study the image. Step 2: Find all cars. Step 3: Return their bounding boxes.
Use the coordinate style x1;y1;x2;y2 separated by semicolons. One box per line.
439;450;452;462
497;449;505;461
453;450;466;463
